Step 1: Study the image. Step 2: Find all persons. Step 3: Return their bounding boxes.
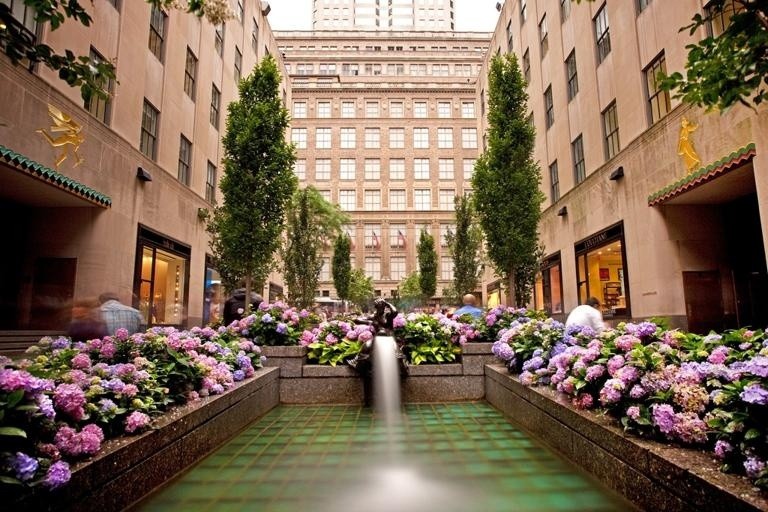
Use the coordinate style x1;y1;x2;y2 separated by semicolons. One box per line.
347;298;407;368
452;294;485;321
223;277;264;329
562;296;608;340
57;291;146;342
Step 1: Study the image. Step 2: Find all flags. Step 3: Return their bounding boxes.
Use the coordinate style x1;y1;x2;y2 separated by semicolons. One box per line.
372;232;379;247
397;231;406;247
